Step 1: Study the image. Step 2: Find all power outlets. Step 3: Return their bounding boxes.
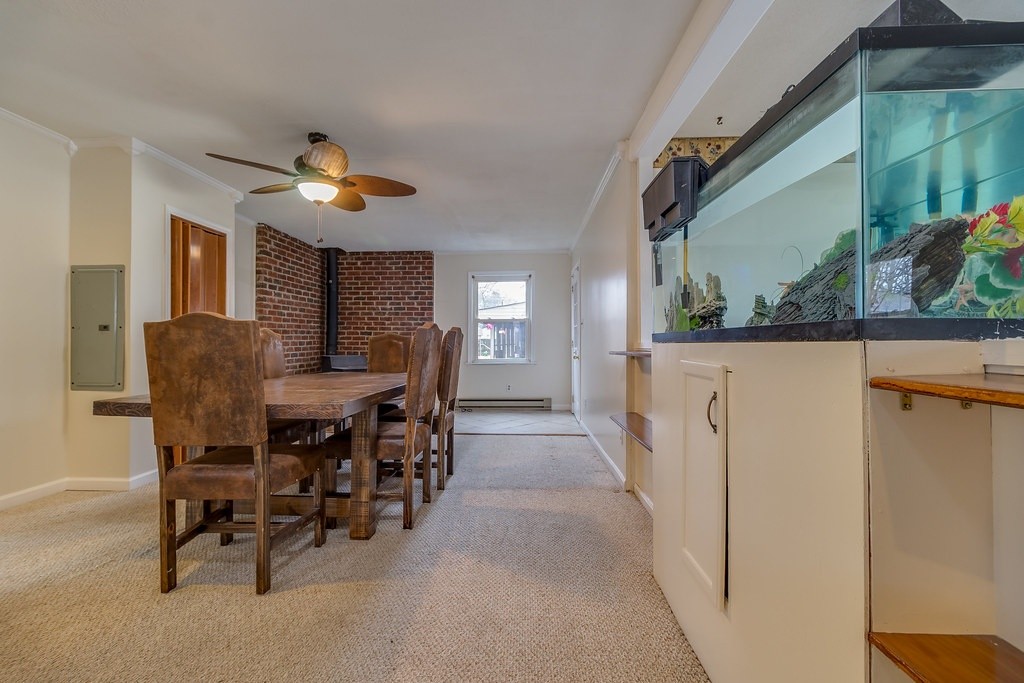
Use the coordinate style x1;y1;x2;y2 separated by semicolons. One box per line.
618;427;623;445
507;384;512;392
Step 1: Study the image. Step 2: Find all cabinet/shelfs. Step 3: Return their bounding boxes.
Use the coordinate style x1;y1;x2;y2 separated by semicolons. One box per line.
608;349;653;453
680;359;730;612
866;372;1024;683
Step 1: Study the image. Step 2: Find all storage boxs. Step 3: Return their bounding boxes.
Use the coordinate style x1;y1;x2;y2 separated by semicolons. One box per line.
651;22;1024;343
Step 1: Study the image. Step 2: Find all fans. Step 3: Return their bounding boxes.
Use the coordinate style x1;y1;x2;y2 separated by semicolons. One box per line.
205;132;418;212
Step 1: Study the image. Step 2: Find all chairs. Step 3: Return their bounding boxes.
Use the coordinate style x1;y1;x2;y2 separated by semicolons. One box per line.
143;312;328;596
377;327;464;490
260;327;319;445
366;331;413;469
323;321;444;530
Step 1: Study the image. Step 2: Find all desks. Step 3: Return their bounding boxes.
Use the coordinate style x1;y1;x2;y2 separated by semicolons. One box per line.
93;371;408;541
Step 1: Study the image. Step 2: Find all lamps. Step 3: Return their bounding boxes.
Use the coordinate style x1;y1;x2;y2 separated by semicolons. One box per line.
292;177;342;206
